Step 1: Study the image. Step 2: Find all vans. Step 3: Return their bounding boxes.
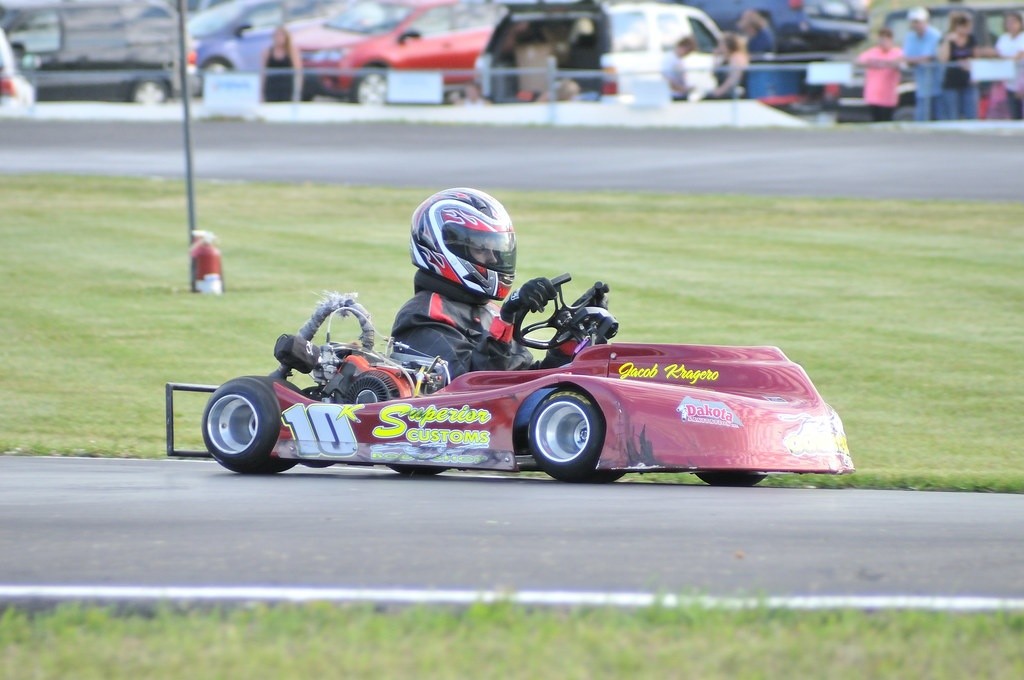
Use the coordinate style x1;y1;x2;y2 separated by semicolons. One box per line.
0;0;204;119
473;0;725;100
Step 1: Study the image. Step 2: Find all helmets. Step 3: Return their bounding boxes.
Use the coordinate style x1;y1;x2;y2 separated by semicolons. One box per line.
409;187;517;301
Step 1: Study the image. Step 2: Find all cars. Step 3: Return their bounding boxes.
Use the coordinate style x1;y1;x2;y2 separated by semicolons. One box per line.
688;0;869;64
188;0;510;102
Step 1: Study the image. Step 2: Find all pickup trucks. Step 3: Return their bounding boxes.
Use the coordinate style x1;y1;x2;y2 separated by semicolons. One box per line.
822;5;1024;123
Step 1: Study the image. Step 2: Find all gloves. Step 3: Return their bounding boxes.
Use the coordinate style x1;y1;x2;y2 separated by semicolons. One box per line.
570;284;609;310
500;277;557;323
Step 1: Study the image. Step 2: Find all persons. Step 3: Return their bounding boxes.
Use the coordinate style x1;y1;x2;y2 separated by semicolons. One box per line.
737;11;776;63
901;7;943;118
390;187;608;396
670;38;719;100
857;28;903;121
258;25;304;101
995;11;1024;117
490;17;579;104
715;33;748;98
937;12;997;121
